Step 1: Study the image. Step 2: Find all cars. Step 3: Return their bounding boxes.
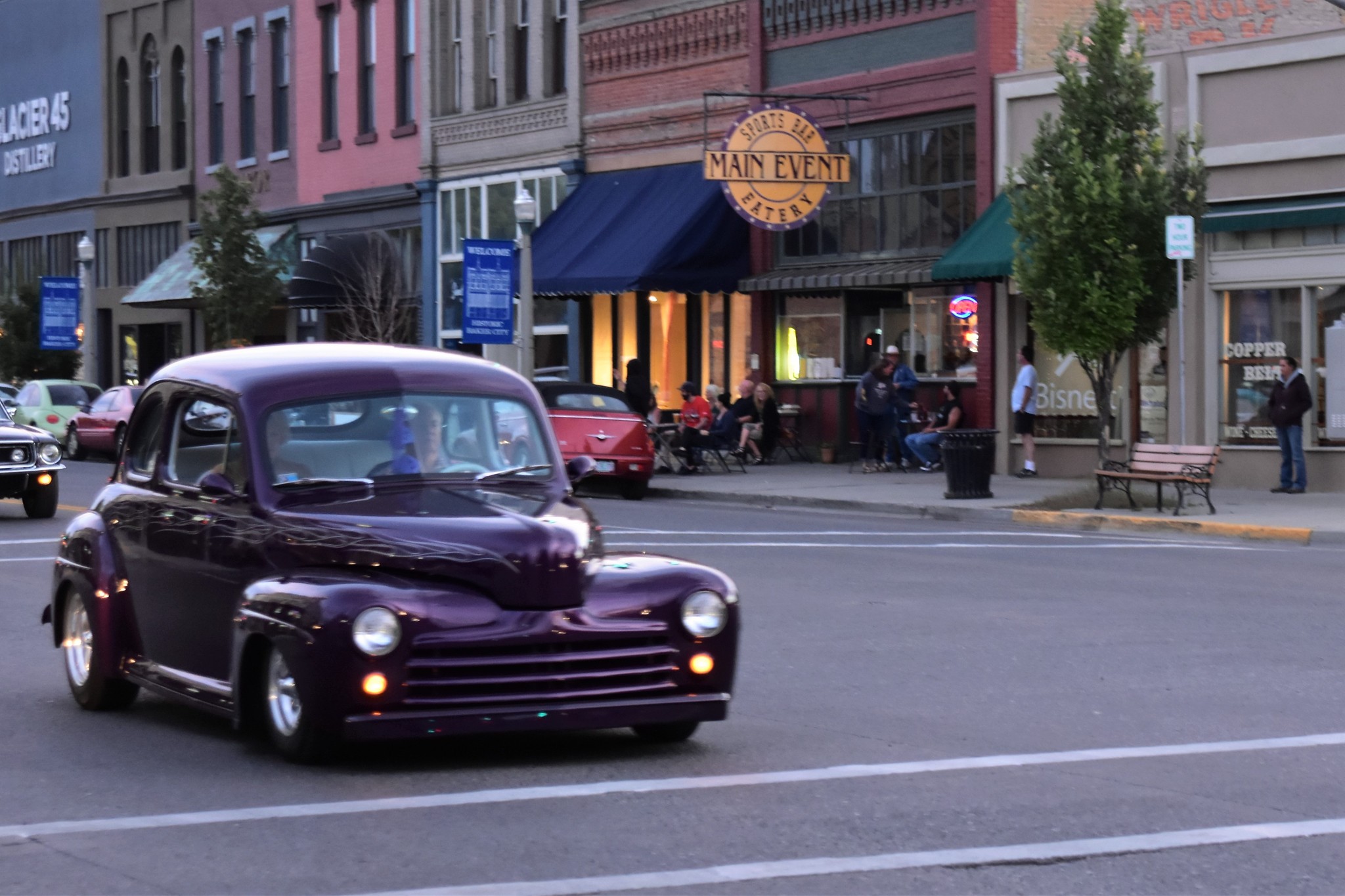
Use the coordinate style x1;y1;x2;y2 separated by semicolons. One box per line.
64;385;144;462
0;383;20;404
43;341;742;766
2;379;105;446
452;379;656;502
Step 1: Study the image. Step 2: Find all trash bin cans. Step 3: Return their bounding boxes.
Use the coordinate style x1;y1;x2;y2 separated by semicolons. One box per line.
936;429;1000;499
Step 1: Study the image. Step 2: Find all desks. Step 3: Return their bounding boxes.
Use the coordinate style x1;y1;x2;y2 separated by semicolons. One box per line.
648;423;696;471
898;420;932;467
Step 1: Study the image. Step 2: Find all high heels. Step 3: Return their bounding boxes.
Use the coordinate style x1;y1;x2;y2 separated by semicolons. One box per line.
919;461;945;472
729;447;747;460
749;456;764;465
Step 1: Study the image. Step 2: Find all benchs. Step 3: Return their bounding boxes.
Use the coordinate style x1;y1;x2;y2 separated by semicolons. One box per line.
1090;442;1221;516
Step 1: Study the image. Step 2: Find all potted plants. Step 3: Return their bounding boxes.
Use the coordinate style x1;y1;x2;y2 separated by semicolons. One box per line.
820;443;835;460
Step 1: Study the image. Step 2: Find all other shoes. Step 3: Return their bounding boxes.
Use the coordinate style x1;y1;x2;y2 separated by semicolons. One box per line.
861;459;915;473
654;466;672;474
1013;467;1038;478
1271;487;1287;493
1288;488;1304;494
677;466;699;475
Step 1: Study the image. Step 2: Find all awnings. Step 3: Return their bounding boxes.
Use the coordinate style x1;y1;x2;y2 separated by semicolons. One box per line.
120;223;296;308
513;159;749;295
931;186;1018;276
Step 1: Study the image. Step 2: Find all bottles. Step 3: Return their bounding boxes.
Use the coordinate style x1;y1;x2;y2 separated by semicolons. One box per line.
910;411;918;420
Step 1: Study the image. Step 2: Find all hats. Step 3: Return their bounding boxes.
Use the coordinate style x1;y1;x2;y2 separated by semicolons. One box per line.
885;345;899;354
677;382;697;394
718;391;732;407
706;384;720;396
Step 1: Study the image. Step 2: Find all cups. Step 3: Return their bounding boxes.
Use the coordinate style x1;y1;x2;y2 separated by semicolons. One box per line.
673;413;680;423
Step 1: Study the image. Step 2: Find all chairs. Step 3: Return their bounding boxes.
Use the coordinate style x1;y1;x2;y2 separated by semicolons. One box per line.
696;419;746;473
844;415;907;474
763;414;806;463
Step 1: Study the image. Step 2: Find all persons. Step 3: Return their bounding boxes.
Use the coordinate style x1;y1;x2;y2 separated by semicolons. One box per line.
733;380;760;452
906;381;963;473
882;345;918;470
1148;345;1168;440
367;402;474;472
1011;346;1039;477
199;410;308;488
612;359;732;472
1269;357;1313;495
729;382;778;465
854;359;917;471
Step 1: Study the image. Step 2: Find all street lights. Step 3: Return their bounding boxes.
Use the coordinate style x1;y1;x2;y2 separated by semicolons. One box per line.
76;235;100;385
513;188;535;381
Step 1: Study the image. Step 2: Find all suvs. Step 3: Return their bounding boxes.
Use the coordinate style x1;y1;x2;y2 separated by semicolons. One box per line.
0;398;67;520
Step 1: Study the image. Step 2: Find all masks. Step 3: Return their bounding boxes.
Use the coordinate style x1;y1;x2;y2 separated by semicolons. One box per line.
682;394;689;400
